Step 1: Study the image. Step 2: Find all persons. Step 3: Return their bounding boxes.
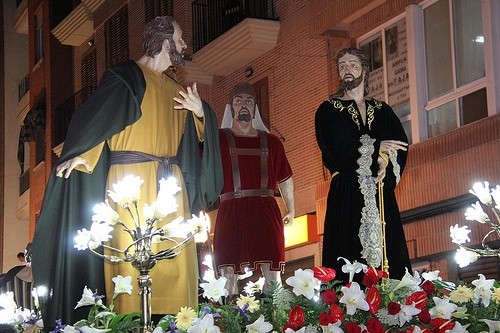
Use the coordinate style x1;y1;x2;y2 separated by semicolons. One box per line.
17;252;26;263
314;47;412;287
31;16;223;328
213;83;295;296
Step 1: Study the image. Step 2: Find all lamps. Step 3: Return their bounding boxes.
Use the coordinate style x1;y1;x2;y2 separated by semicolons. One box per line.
73;174;211;327
449;180;500;268
0;286;49;325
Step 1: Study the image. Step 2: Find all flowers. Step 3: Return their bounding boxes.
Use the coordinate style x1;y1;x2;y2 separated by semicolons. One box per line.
54;258;500;333
